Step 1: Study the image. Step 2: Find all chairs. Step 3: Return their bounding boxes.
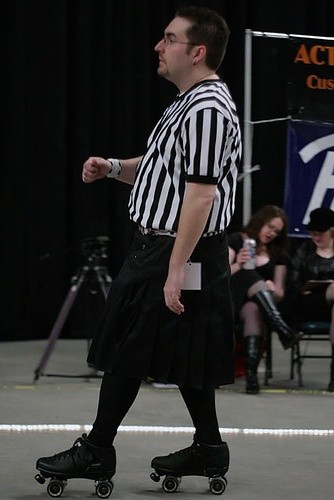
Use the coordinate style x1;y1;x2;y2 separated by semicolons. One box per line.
232;260;331;388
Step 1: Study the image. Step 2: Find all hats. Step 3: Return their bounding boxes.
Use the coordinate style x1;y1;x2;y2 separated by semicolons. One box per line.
306;208;334;233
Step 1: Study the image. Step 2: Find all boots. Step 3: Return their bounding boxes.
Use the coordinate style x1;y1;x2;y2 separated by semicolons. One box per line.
242;334;264;394
328;343;334;392
250;289;304;350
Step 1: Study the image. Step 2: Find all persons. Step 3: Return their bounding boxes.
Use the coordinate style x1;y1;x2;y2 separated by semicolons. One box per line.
229;205;301;395
287;207;334;391
36;7;242;480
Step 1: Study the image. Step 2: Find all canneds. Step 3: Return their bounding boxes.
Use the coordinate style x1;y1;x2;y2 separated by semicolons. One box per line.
243;238;256;270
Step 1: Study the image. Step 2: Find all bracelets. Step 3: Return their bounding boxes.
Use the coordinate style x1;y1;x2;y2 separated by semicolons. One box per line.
106;158;121;178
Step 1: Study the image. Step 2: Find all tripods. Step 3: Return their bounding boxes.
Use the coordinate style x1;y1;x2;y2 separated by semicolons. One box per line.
29;236;153;385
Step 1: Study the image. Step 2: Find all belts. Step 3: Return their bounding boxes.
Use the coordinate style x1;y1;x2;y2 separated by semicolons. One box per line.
138;223;222;238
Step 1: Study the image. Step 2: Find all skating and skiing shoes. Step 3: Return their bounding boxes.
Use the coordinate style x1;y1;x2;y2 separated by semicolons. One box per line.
34;433;117;499
150;439;230;495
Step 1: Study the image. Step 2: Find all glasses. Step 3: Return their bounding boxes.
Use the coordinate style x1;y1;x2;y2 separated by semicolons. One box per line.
163;36;208;47
266;222;280;236
